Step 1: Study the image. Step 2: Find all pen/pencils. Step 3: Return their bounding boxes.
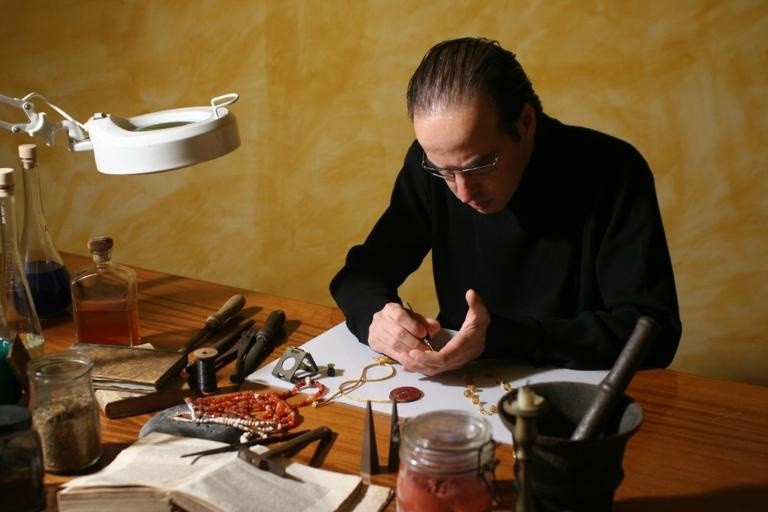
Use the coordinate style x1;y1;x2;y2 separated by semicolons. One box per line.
402;301;435;351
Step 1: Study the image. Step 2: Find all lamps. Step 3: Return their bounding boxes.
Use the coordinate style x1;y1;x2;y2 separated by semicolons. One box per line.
1;87;243;177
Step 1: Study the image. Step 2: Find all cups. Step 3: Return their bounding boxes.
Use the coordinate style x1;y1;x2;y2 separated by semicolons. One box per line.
499;380;646;512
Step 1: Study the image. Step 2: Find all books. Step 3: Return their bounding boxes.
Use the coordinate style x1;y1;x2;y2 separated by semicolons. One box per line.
67;340;187;393
54;432;396;511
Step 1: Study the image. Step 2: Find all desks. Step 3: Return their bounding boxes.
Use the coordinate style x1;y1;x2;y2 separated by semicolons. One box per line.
0;244;768;511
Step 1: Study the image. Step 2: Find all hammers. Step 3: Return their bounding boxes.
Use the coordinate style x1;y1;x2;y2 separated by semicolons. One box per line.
237;425;332;478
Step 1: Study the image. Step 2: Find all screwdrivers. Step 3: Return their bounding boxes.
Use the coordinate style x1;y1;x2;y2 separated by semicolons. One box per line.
177;294;246;353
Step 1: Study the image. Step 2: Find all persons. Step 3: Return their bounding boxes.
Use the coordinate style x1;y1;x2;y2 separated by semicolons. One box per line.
326;34;684;379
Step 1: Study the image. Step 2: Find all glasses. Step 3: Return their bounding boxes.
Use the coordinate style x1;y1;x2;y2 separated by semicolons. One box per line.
421;152;501;180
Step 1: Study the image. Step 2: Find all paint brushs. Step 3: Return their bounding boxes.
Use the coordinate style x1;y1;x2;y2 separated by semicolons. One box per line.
180;429;311;458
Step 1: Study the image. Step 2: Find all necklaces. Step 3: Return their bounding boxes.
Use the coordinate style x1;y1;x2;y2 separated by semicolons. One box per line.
178;380;327;444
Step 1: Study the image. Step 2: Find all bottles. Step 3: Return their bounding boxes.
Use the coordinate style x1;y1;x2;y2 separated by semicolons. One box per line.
73;237;140;344
398;410;495;511
0;166;45;359
13;141;74;323
0;337;104;512
